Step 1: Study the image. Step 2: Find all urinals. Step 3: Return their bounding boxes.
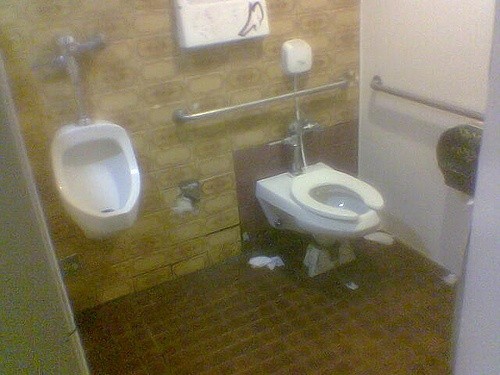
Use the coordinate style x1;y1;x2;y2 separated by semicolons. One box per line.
51;119;142;241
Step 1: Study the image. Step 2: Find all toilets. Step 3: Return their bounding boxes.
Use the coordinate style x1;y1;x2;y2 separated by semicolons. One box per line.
256;161;383;278
